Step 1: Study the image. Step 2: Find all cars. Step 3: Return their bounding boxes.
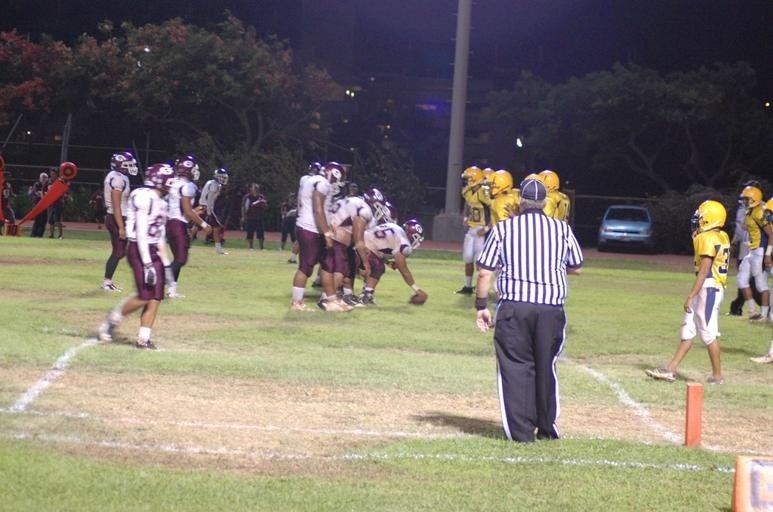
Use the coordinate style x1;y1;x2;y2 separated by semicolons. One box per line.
596;205;656;254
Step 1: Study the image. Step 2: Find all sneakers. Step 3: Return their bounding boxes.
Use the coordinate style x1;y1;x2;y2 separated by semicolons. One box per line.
289;298;316;313
359;294;379;308
645;367;678;383
748;314;769;322
101;281;122;293
95;328;118;344
749;356;773;364
134;339;161;352
699;378;725;387
316;293;361;313
165;292;185;299
286;260;297;265
456;285;474;295
215;251;228;256
741;312;761;321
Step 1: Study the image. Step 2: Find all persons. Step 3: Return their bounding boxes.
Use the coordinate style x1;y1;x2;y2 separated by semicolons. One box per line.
279;191;296;250
2;180;16;225
475;177;585;444
101;151;229;349
454;166;572;294
644;200;731;386
27;167;68;239
291;160;425;312
240;182;268;251
724;180;773;365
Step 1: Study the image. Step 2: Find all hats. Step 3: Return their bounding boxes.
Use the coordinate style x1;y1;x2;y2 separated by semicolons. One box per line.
519;178;546;200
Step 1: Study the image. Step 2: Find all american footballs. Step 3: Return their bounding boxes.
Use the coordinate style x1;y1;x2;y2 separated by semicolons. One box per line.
410;294;426;305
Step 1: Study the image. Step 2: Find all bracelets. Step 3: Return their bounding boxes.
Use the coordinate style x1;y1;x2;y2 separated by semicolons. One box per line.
475;296;488;311
200;222;208;229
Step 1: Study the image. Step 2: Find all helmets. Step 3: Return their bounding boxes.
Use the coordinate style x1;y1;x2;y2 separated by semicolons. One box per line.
363;187;385;217
694;200;726;231
403;219;426;249
307;161;321;176
215;168;229;185
740;185;763;208
460;165;485;190
173;154;200;181
109;151;138;177
481;168;494;179
322;162;346;190
143;162;175;195
539;170;561;190
380;202;398;225
488;169;515;197
